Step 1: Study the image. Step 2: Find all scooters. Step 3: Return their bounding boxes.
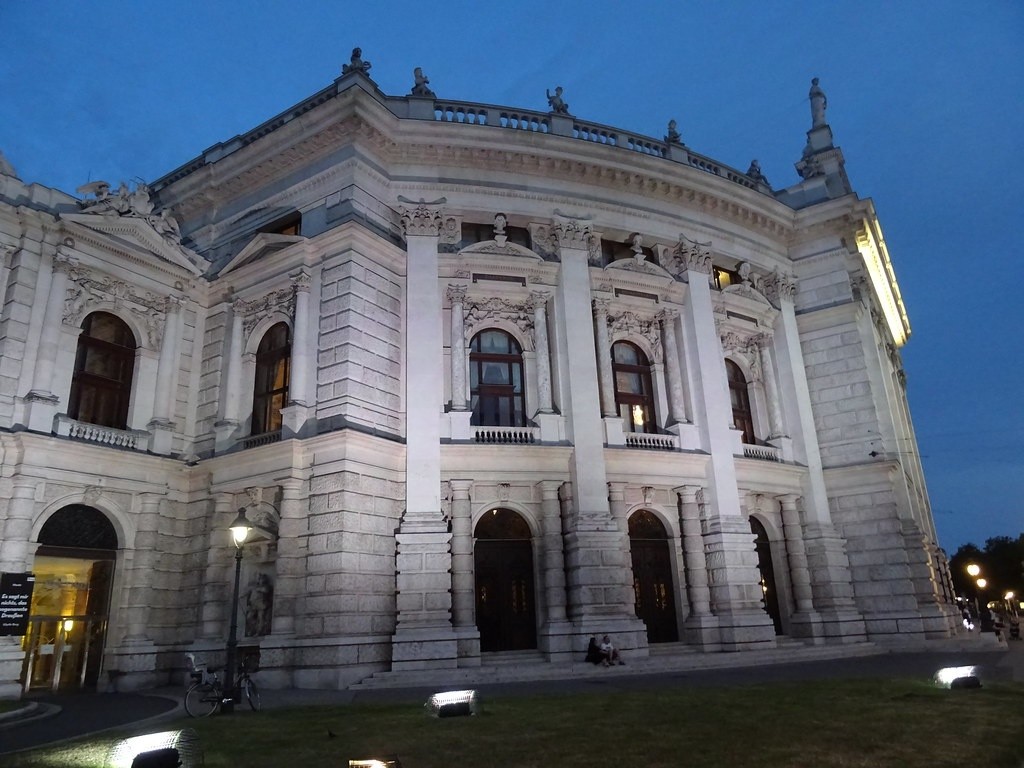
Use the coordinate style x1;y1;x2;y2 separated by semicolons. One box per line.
1009;619;1020;640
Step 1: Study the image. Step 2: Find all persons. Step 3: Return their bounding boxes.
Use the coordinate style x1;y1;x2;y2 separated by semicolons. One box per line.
244;294;294;332
664;120;685;146
64;280;162;351
585;636;625;668
443;218;459;242
492;213;506;234
809;77;827;128
414;67;431;97
630;235;643;253
547;86;571;115
746;159;771;186
963;605;972;631
342;47;372;71
872;305;906;391
238;574;273;637
78;185;189;259
720;332;762;381
606;311;662;359
463;297;534;350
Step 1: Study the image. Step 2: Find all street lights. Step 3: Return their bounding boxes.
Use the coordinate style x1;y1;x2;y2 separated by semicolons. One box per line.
219;507;254;715
967;564;982;625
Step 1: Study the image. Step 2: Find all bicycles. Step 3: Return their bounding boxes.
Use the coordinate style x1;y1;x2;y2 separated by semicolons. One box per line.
183;652;261;718
